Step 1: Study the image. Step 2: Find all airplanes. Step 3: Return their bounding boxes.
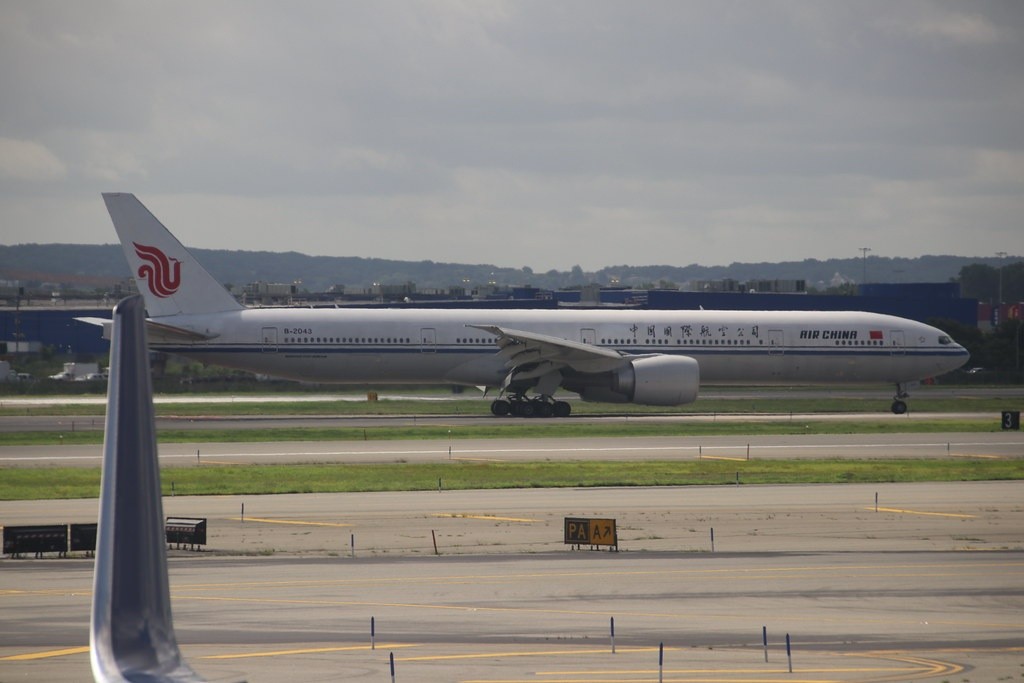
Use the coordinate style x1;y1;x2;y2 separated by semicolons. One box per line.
70;191;971;416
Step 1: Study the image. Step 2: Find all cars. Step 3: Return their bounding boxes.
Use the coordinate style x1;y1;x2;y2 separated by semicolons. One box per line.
18;361;109;383
